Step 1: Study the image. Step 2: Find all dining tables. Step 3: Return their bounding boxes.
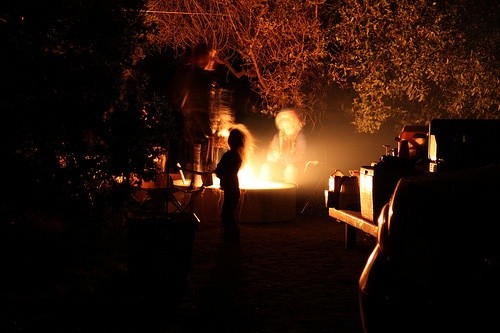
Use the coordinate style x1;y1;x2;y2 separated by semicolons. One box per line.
328;207;379;253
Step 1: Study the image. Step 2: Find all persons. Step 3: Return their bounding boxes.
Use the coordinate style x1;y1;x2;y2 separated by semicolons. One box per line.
214;128;246;253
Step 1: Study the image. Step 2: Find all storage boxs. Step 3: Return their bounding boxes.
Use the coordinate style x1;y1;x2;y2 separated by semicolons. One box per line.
360;165;393;223
